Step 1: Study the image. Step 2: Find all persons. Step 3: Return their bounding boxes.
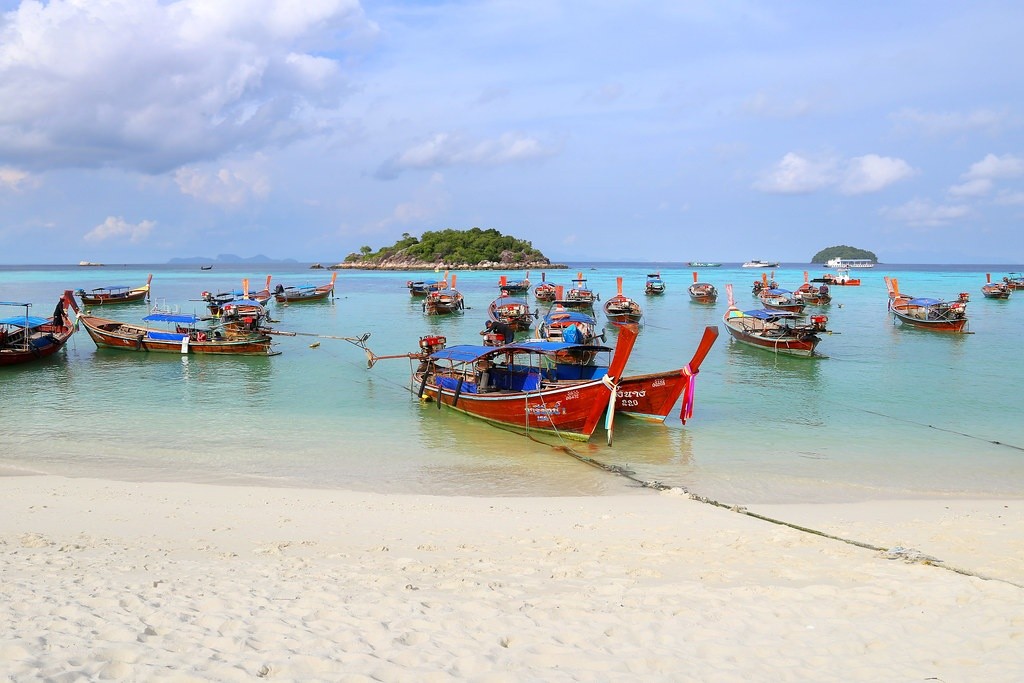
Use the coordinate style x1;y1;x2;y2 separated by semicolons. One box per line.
479;320;514;367
53;297;67;337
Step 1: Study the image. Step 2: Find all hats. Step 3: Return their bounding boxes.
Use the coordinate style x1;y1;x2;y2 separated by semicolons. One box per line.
485;320;491;329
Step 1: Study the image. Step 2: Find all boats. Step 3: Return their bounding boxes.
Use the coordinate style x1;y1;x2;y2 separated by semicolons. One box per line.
534;271;560;303
200;264;213;270
534;300;601;366
722;270;833;358
496;326;719;424
688;262;722;267
1002;273;1024;290
883;276;971;332
645;271;666;296
487;289;533;333
420;274;464;316
741;258;781;268
810;264;861;286
271;272;337;304
65;275;296;357
687;271;719;306
981;273;1013;300
367;320;641;444
406;270;449;297
72;273;153;307
565;272;597;310
497;269;533;294
603;276;643;327
0;289;75;368
823;256;875;268
